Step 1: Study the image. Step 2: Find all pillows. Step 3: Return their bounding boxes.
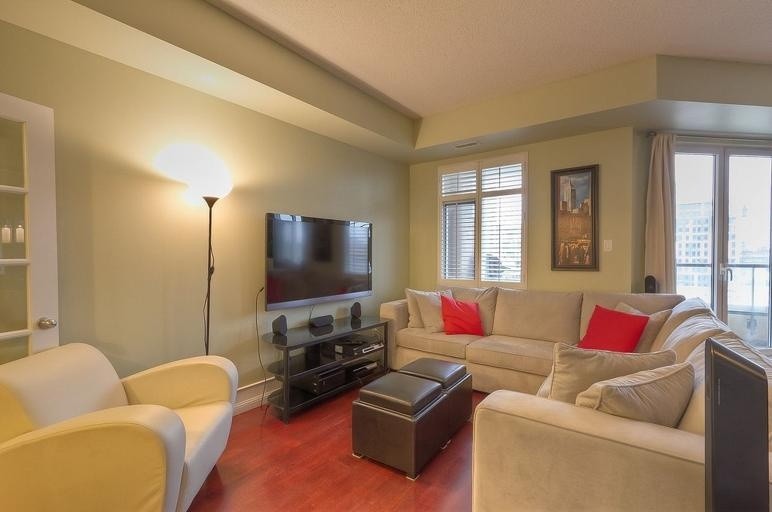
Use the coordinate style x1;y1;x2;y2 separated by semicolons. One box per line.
579;304;649;353
576;363;695;427
612;300;671;353
441;295;484;336
404;287;440;328
415;291;453;332
549;337;676;403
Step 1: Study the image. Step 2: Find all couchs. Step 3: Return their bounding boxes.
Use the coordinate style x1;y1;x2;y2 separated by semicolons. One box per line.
379;284;771;512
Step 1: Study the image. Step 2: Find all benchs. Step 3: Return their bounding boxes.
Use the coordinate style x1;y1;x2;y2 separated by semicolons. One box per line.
353;356;473;481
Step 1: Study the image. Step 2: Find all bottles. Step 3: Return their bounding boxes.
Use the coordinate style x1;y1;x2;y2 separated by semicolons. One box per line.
1;224;11;243
15;224;25;242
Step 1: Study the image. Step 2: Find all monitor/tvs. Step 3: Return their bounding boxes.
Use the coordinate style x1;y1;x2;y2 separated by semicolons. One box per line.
264;211;373;312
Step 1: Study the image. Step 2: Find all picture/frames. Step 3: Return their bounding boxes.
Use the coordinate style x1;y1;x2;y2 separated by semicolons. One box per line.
550;165;600;273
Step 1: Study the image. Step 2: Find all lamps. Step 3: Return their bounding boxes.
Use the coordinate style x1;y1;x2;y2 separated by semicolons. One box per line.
197;154;237;355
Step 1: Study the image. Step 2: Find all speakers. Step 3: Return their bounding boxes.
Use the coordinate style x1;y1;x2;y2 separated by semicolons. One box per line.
351;302;361;318
309;315;334;327
644;275;656;293
272;315;287;336
705;337;769;512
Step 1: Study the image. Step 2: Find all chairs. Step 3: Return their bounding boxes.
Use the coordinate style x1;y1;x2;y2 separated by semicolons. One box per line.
0;340;239;512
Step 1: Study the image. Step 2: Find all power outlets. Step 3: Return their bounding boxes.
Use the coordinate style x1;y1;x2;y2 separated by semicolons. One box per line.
604;239;618;254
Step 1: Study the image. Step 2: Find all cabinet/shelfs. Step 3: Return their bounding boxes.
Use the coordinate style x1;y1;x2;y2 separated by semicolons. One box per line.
260;316;390;423
